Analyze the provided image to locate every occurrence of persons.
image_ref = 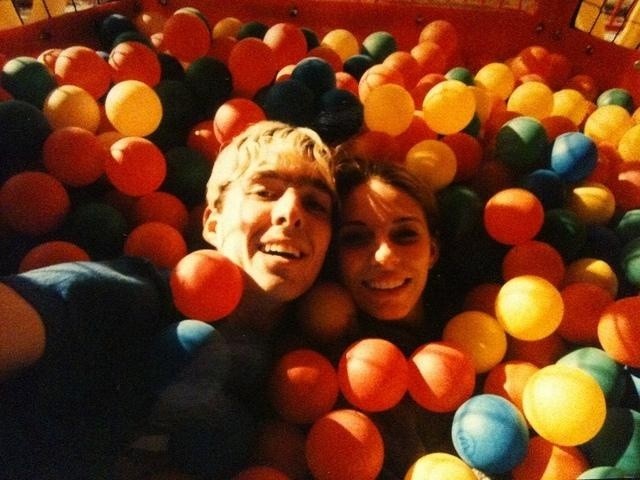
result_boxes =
[327,157,460,364]
[1,121,338,480]
